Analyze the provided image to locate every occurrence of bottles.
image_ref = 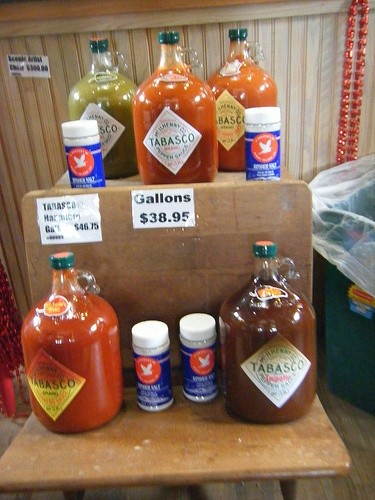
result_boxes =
[131,320,173,412]
[244,107,282,180]
[208,28,278,172]
[178,313,219,403]
[67,37,139,179]
[61,120,106,188]
[132,31,217,184]
[218,241,318,424]
[21,251,122,434]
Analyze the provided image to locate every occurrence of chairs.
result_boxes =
[0,180,352,500]
